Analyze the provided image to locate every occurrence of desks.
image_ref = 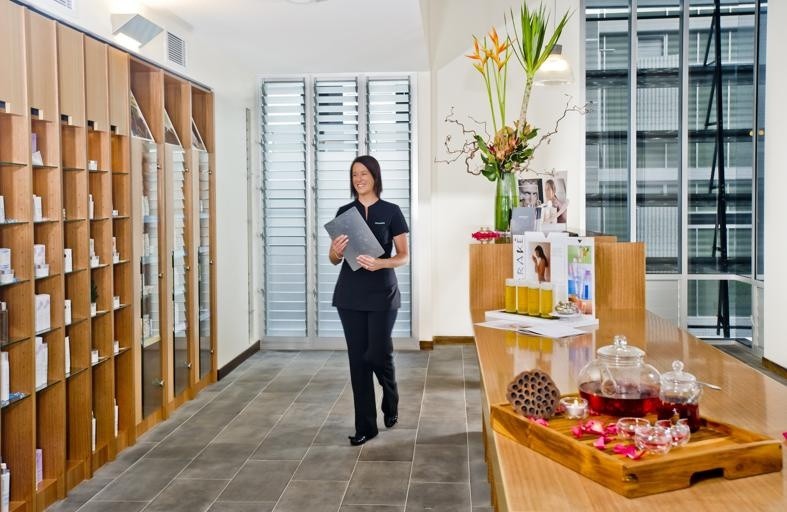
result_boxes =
[471,308,786,510]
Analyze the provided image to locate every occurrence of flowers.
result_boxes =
[434,3,596,181]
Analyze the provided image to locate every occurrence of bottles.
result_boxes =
[577,334,721,434]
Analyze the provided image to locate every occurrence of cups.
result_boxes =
[560,397,588,420]
[618,419,691,452]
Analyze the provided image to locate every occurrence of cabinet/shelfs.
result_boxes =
[1,1,216,511]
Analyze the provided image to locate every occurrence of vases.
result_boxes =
[496,172,518,244]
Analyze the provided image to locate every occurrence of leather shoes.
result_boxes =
[347,431,368,445]
[384,411,398,428]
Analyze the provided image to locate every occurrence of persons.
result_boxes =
[545,179,561,208]
[329,154,411,446]
[532,245,546,281]
[519,179,540,207]
